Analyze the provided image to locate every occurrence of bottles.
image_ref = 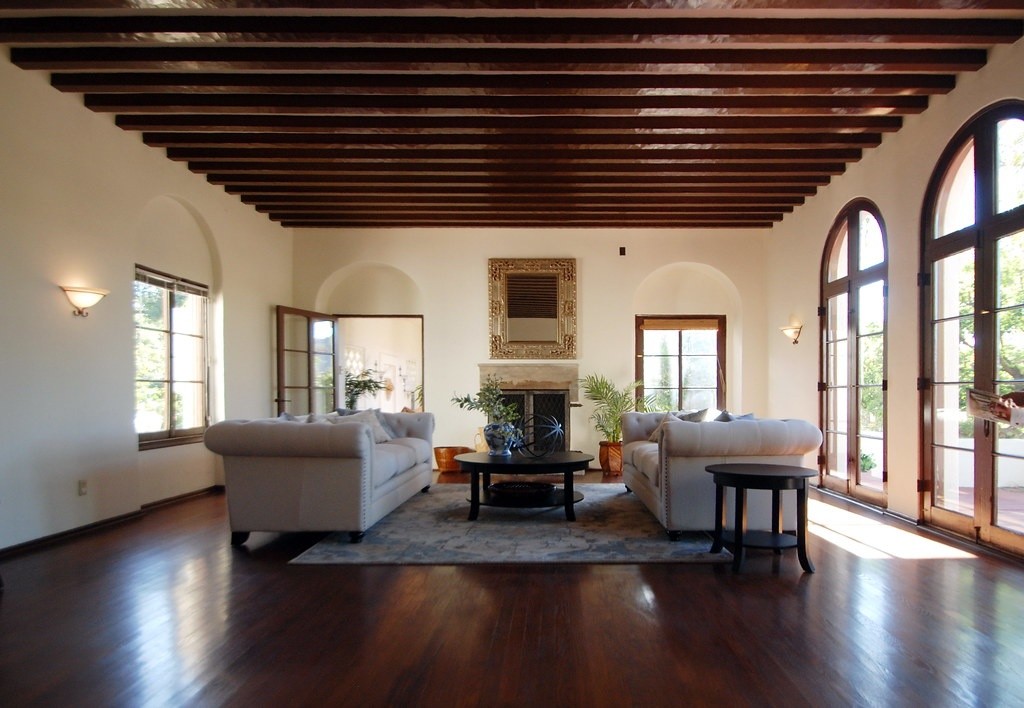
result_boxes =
[475,427,489,452]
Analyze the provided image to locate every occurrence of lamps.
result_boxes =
[58,284,110,318]
[778,326,805,345]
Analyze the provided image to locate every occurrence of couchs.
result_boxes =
[621,412,823,542]
[203,412,436,546]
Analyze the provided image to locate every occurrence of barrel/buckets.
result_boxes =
[434,447,468,471]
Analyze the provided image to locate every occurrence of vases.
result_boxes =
[484,414,516,456]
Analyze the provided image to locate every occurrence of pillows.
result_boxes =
[279,408,397,443]
[649,408,755,443]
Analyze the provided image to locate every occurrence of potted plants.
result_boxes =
[577,373,671,476]
[861,454,877,481]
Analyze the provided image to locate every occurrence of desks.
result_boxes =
[454,451,595,522]
[705,463,819,574]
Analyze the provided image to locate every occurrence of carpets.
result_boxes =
[288,483,733,564]
